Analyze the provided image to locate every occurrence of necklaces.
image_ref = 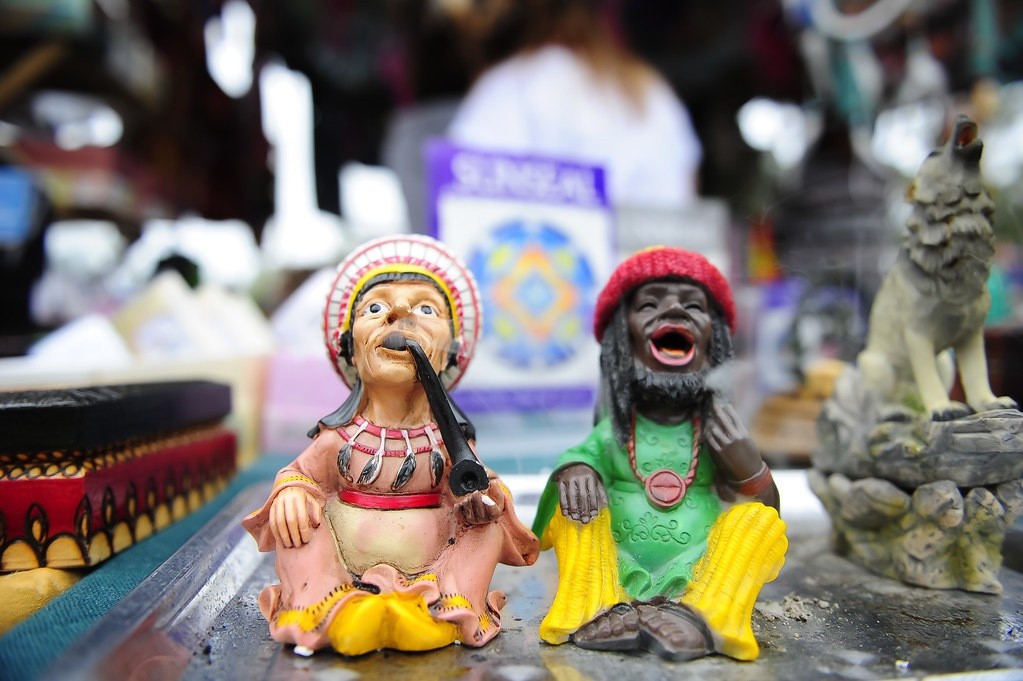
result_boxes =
[626,401,703,511]
[333,413,450,489]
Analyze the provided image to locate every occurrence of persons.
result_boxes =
[241,233,542,654]
[532,246,790,662]
[452,0,704,204]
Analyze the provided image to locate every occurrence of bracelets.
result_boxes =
[723,461,773,497]
